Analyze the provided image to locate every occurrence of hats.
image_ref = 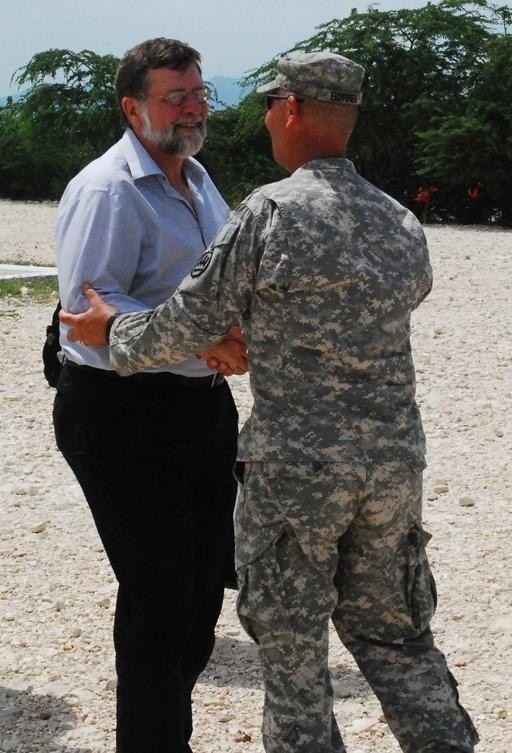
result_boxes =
[257,52,365,105]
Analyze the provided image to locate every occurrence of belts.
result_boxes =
[57,352,224,388]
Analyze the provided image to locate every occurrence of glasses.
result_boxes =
[141,85,215,105]
[264,91,306,110]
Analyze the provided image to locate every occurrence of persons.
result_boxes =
[42,34,248,752]
[57,51,481,753]
